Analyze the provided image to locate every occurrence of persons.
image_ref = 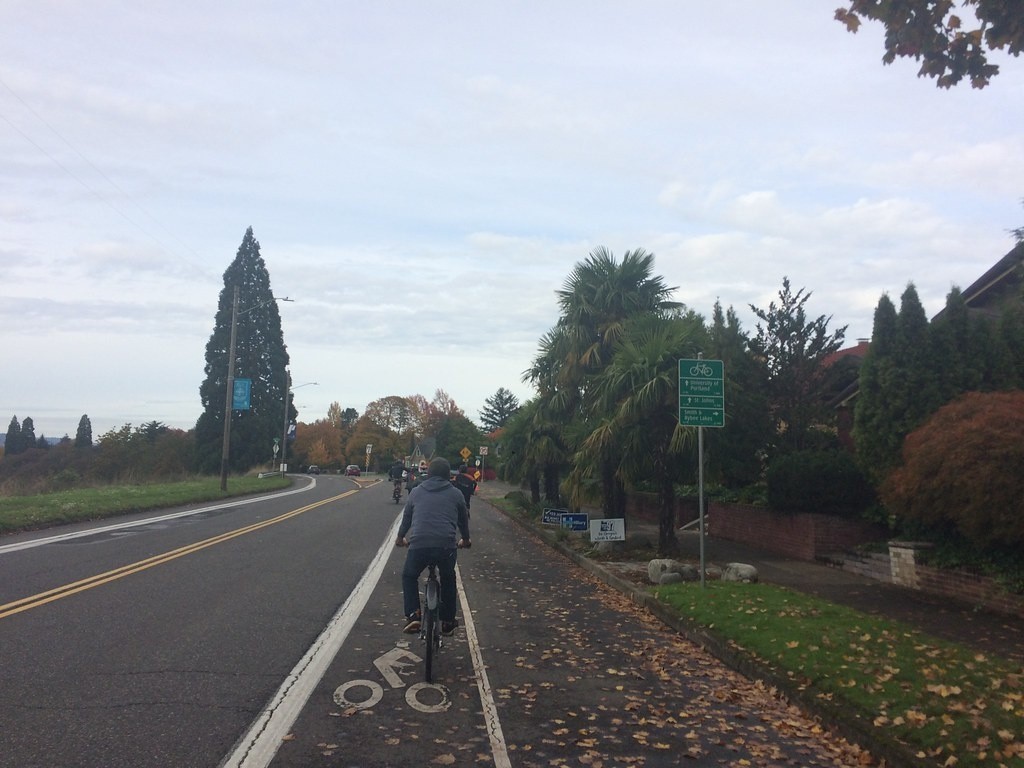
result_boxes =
[395,458,472,635]
[388,460,477,517]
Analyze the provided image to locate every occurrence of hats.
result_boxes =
[459,465,468,473]
[428,457,450,480]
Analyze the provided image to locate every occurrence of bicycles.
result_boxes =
[394,537,472,684]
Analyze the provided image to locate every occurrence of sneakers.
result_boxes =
[402,611,421,633]
[442,618,458,635]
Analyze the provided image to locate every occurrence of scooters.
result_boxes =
[388,475,407,504]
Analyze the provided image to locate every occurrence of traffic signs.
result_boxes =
[678,359,725,429]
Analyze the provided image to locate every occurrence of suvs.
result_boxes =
[307,465,320,476]
[344,465,361,477]
